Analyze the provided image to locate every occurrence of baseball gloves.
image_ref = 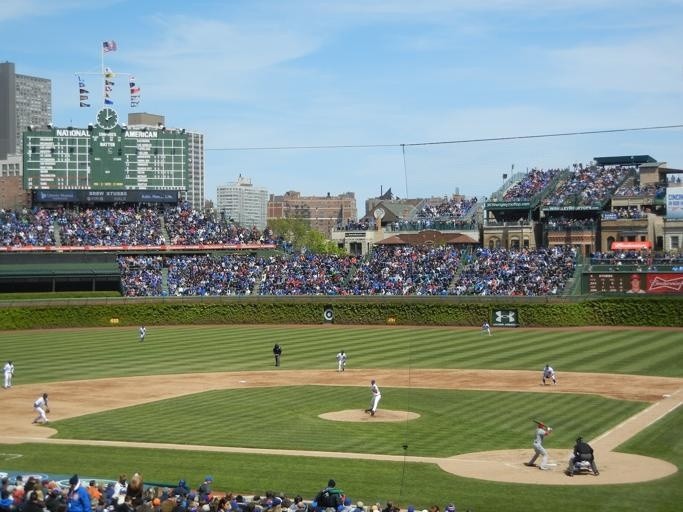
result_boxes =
[46,409,50,413]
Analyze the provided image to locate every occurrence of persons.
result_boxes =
[367,380,381,415]
[139,323,146,341]
[481,322,491,336]
[3,360,15,388]
[32,393,49,424]
[336,350,346,372]
[591,245,683,271]
[337,196,478,230]
[0,200,273,246]
[567,436,600,476]
[493,163,680,230]
[626,275,646,293]
[273,344,281,367]
[528,422,553,470]
[543,364,556,385]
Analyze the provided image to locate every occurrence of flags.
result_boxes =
[78,74,141,108]
[103,40,118,53]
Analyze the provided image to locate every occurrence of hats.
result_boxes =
[205,476,213,481]
[70,473,79,484]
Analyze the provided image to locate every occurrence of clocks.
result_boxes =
[95,106,119,131]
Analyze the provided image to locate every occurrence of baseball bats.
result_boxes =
[532,419,548,428]
